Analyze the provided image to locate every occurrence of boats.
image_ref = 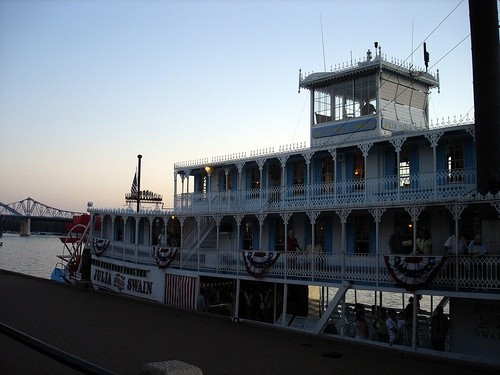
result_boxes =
[51,41,499,374]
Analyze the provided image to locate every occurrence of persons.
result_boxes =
[468,233,488,257]
[287,229,322,255]
[443,227,467,256]
[264,287,279,322]
[355,294,449,352]
[158,227,169,247]
[389,224,413,254]
[416,227,433,256]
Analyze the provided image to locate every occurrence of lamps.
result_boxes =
[171,215,175,219]
[355,169,358,175]
[256,179,258,184]
